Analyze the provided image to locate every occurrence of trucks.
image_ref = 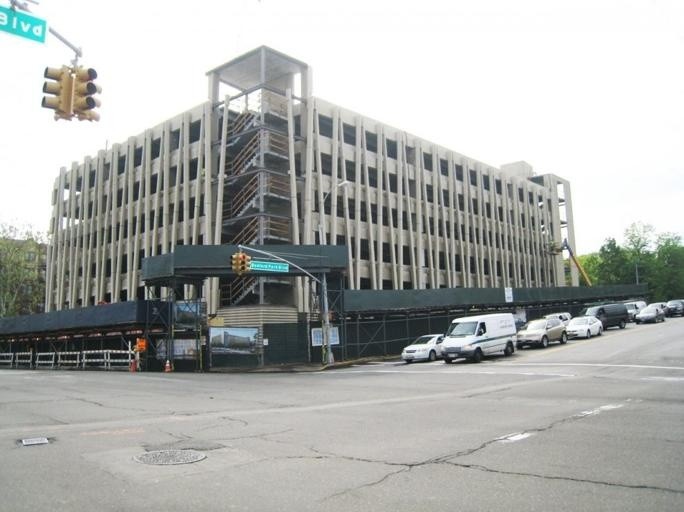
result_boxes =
[584,302,630,331]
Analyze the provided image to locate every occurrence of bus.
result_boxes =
[565,315,604,340]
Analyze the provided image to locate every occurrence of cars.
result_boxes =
[635,299,684,325]
[400,333,444,364]
[515,317,568,350]
[544,312,571,326]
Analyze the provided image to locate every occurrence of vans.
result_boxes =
[440,312,518,365]
[624,300,647,321]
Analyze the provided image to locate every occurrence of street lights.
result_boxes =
[318,179,352,365]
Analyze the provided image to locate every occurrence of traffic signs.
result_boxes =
[0,3,49,44]
[249,261,289,273]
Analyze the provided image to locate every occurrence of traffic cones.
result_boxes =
[164,358,173,373]
[129,358,138,373]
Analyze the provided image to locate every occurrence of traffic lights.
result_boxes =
[39,62,72,122]
[79,83,104,123]
[237,253,246,275]
[245,254,252,274]
[71,64,97,115]
[229,252,238,272]
